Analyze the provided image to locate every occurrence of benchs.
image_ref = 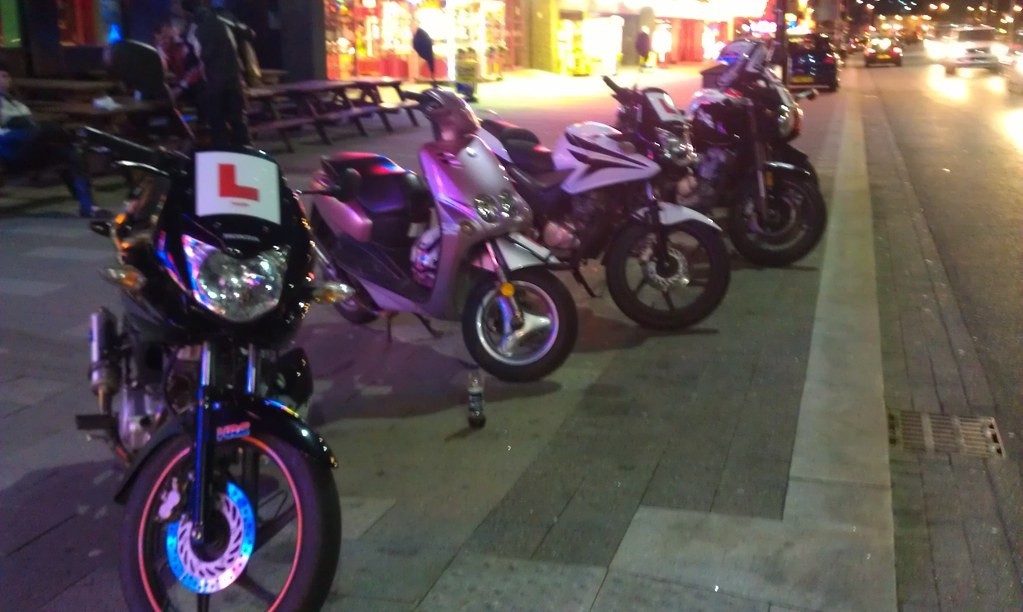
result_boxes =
[90,99,425,156]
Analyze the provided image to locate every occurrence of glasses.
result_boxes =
[689,88,735,144]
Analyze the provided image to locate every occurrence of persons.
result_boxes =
[150,0,257,154]
[637,25,653,69]
[0,69,115,218]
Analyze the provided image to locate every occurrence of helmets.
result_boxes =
[411,228,438,290]
[718,38,766,88]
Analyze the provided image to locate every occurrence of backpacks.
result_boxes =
[158,15,208,104]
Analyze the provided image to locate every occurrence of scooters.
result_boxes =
[308,27,578,384]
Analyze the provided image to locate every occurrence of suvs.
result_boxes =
[942,26,1008,77]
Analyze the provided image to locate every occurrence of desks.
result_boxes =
[11,73,404,183]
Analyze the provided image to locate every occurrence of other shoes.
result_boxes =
[80,204,114,220]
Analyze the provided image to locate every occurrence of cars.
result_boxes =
[784,35,843,94]
[862,35,904,69]
[832,27,936,65]
[1003,31,1023,94]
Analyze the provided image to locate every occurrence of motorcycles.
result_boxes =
[660,40,829,266]
[477,75,734,333]
[73,1,365,612]
